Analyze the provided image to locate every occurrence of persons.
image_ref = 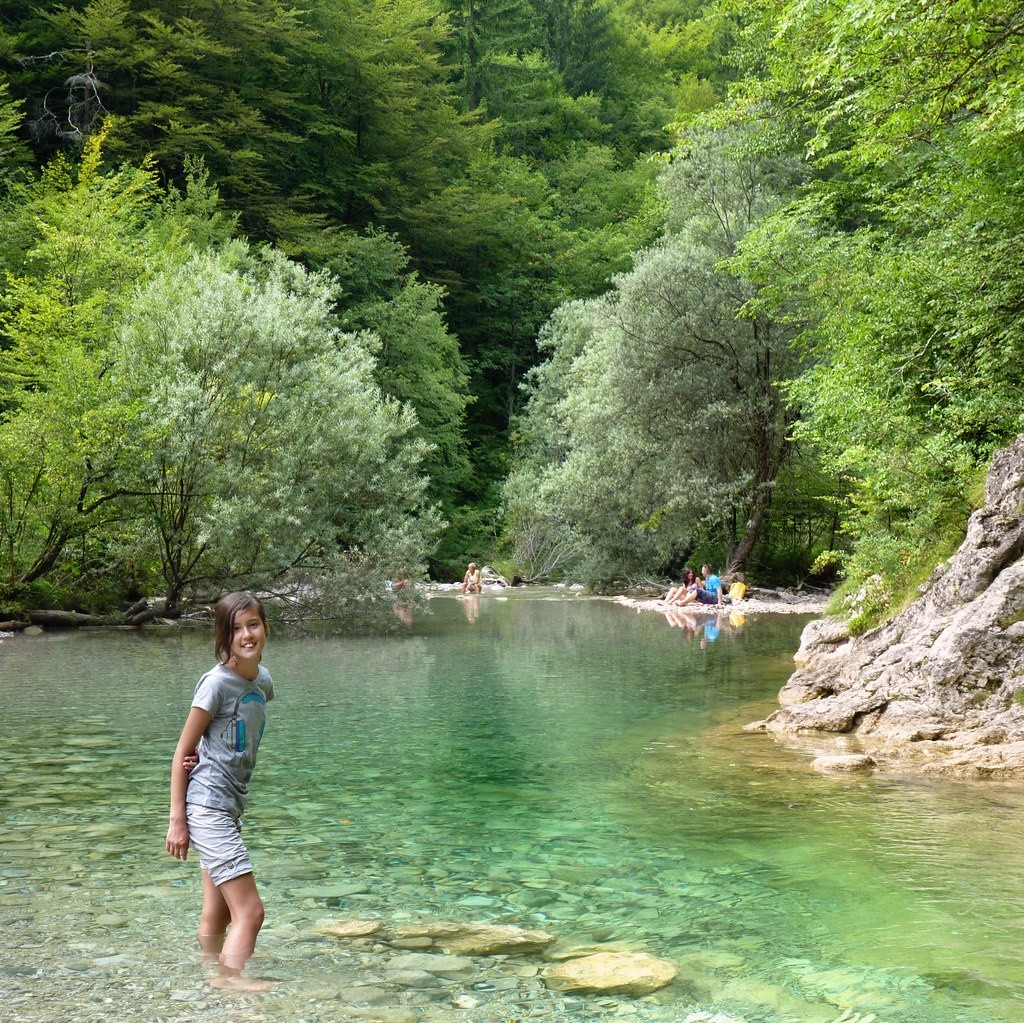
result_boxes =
[460,562,482,595]
[165,591,280,992]
[657,563,723,609]
[389,567,409,592]
